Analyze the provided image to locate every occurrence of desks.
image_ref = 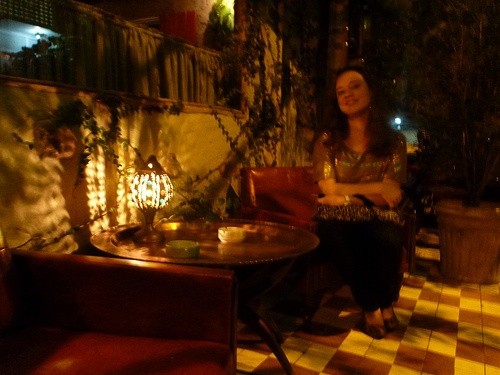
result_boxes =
[89,217,320,375]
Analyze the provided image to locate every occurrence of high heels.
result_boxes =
[362,307,398,338]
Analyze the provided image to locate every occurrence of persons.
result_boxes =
[310,63,408,339]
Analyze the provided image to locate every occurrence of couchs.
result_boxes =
[0,245,235,375]
[240,165,416,317]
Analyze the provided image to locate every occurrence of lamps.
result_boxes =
[128,156,174,243]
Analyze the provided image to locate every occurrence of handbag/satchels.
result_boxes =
[316,194,404,226]
